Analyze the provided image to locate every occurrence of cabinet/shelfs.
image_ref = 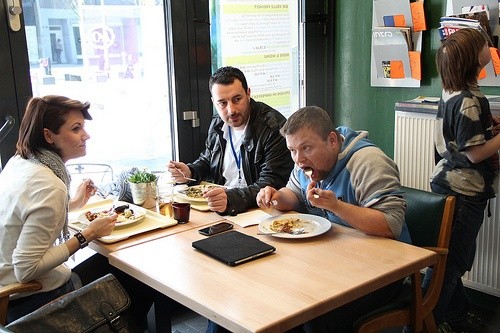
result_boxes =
[393,96,500,300]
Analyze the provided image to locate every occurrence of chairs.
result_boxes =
[354,186,458,333]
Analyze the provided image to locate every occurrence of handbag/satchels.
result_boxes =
[5,273,131,333]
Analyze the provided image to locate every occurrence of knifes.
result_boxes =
[266,199,277,206]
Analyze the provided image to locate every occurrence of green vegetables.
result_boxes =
[128,170,155,184]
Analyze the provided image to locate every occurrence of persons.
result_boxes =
[167,64,294,216]
[418,27,500,331]
[0,94,157,332]
[256,106,414,247]
[56,38,63,63]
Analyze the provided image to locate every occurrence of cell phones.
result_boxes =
[199,222,233,236]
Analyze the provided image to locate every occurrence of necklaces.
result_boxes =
[228,124,242,184]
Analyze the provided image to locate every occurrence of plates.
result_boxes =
[258,213,331,238]
[179,184,229,201]
[78,204,146,226]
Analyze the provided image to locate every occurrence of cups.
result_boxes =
[171,201,189,225]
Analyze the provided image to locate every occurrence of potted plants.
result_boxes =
[129,171,158,208]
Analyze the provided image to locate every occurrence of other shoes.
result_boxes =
[437,310,497,333]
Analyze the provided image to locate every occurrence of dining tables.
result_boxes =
[65,181,266,254]
[110,206,437,333]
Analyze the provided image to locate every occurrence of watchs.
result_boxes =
[74,232,89,249]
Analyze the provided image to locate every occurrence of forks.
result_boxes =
[172,165,197,183]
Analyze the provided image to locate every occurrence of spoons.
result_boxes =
[256,228,303,237]
[303,167,320,200]
